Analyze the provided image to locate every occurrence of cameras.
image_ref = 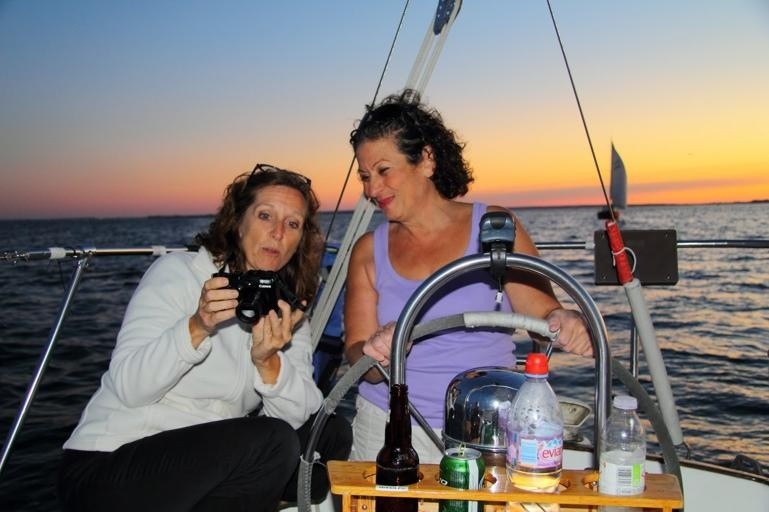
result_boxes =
[210,270,296,325]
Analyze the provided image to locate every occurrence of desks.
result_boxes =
[327,457,683,512]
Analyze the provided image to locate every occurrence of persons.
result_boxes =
[61,164,352,512]
[344,88,595,464]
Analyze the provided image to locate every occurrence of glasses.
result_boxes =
[244,164,311,197]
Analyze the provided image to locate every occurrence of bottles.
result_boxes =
[596,395,646,512]
[375,382,419,512]
[504,350,564,512]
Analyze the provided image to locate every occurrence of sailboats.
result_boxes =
[598,142,627,220]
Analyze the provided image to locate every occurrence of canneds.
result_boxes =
[438,442,485,512]
